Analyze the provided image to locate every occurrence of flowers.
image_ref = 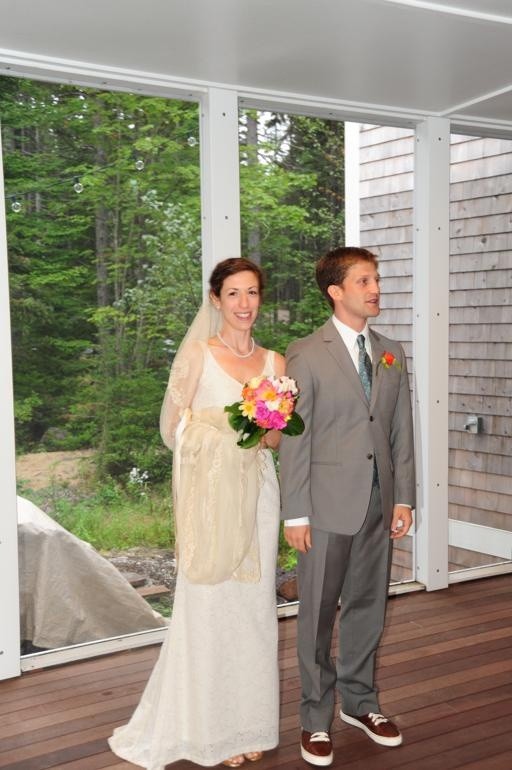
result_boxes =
[223,374,306,447]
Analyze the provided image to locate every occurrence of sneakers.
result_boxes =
[300,730,335,767]
[338,705,402,748]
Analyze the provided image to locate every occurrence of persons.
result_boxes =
[107,259,296,770]
[276,247,418,767]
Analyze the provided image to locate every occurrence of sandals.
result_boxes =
[222,752,262,767]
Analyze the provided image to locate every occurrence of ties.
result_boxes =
[354,335,372,400]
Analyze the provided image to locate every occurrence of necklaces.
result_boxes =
[216,331,260,361]
